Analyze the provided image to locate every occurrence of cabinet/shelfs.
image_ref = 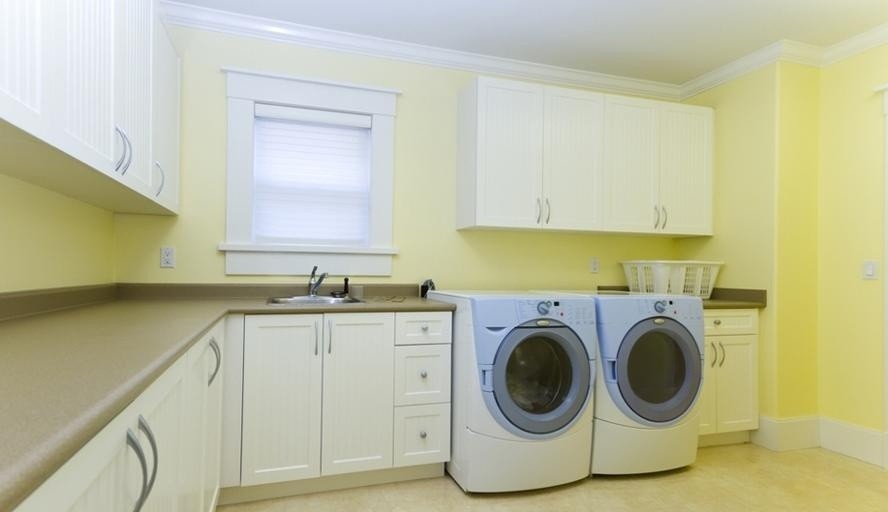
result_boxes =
[0,0,153,201]
[10,359,184,512]
[239,311,394,487]
[394,311,452,469]
[454,75,601,236]
[602,94,713,236]
[185,318,226,512]
[699,308,759,437]
[153,13,181,215]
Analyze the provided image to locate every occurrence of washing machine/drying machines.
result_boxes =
[594,290,704,478]
[427,291,596,494]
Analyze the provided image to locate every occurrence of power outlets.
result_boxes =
[159,247,177,268]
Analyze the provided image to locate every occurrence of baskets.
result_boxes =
[618,258,725,301]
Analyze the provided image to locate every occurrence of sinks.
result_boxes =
[265,295,365,305]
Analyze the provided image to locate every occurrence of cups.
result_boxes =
[349,285,364,300]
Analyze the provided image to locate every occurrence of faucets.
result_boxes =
[307,264,328,295]
